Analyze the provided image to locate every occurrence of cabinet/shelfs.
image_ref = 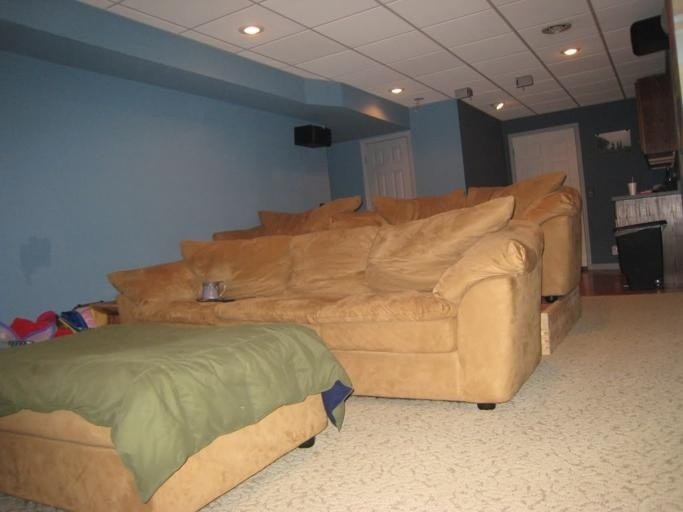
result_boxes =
[634,73,678,170]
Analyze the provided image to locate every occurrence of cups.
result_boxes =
[628,181,637,196]
[202,280,226,299]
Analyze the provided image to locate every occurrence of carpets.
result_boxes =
[3,292,683,512]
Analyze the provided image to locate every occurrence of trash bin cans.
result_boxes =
[612,220,667,290]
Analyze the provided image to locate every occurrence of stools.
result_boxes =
[0,318,356,512]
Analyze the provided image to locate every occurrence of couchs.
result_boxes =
[213,186,584,303]
[94,220,543,410]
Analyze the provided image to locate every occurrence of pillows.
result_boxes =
[178,173,567,298]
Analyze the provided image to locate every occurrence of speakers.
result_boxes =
[294,125,331,147]
